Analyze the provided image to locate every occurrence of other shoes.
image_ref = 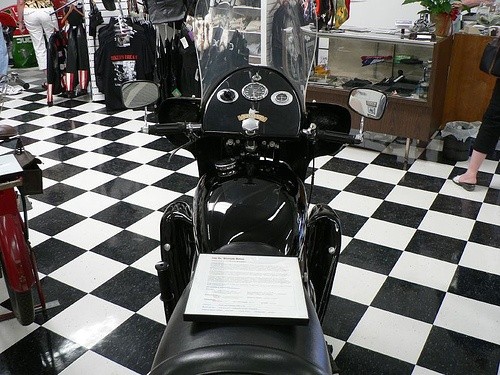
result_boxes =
[42,82,48,89]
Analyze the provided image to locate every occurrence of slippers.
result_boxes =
[452,175,475,191]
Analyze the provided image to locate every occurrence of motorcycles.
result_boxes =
[120,0,389,375]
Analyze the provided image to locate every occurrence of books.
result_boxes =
[183,253,310,326]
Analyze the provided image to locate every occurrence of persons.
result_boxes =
[18,0,60,90]
[452,37,500,191]
[0,21,24,96]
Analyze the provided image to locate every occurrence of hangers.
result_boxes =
[49,0,300,57]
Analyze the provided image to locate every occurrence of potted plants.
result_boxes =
[401,0,471,37]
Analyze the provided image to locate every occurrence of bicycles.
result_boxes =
[0,124,48,326]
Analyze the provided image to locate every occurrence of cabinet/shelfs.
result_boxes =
[439,32,500,139]
[301,23,453,142]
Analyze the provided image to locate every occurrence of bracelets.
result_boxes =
[18,20,23,24]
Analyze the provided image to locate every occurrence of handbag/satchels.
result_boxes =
[479,36,500,77]
